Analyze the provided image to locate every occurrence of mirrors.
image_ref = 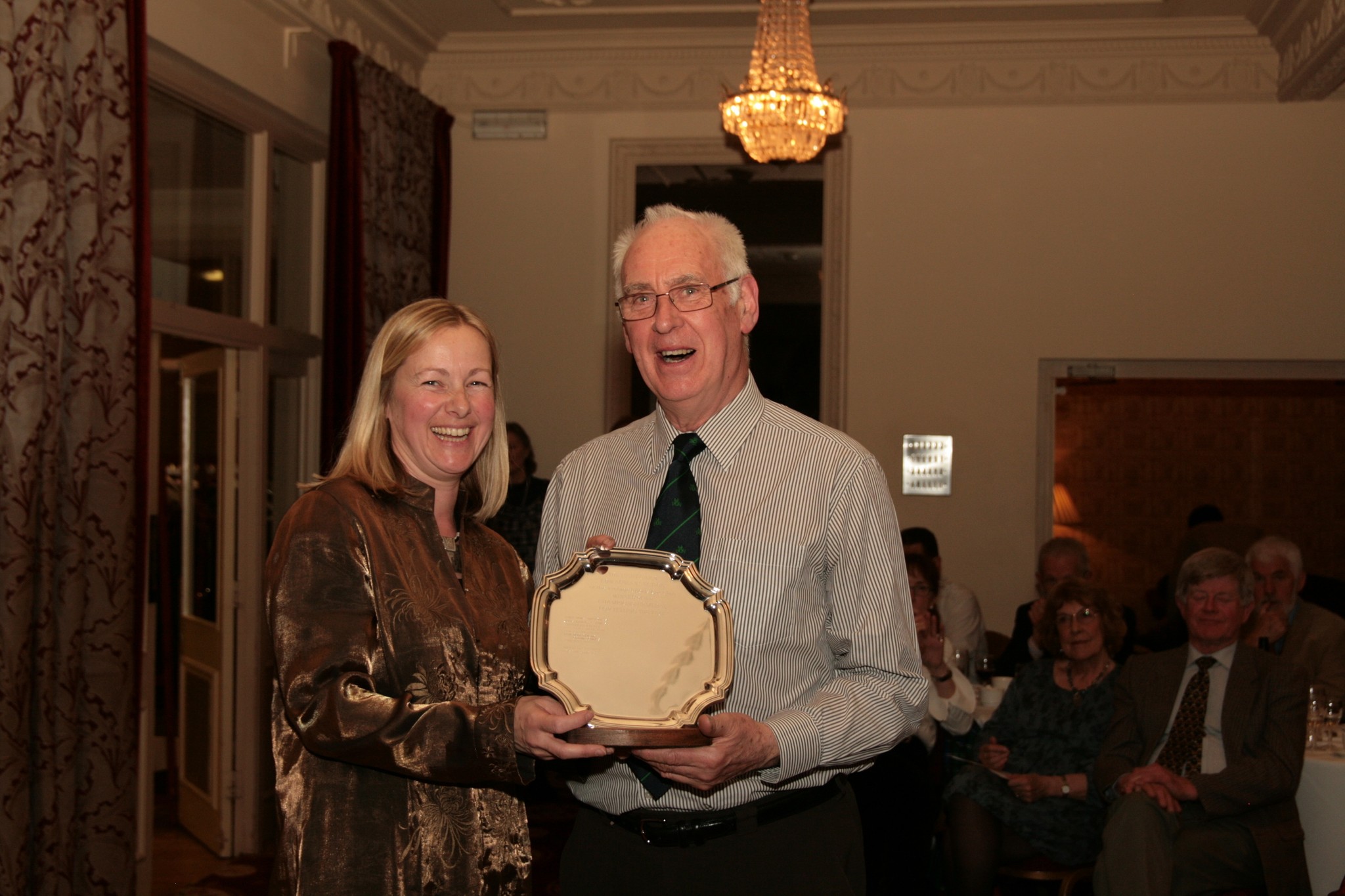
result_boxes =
[1033,356,1344,629]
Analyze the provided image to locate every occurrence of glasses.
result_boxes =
[614,276,739,322]
[1053,607,1097,626]
[908,583,932,596]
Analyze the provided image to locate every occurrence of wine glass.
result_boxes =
[974,652,997,688]
[948,647,970,677]
[1306,683,1343,756]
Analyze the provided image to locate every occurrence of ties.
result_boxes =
[625,433,706,801]
[1155,657,1218,778]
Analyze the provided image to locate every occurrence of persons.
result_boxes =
[264,295,615,894]
[469,417,554,576]
[885,499,1343,896]
[532,204,929,896]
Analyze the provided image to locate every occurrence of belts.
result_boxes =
[603,778,841,848]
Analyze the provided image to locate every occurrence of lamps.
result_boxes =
[718,0,848,164]
